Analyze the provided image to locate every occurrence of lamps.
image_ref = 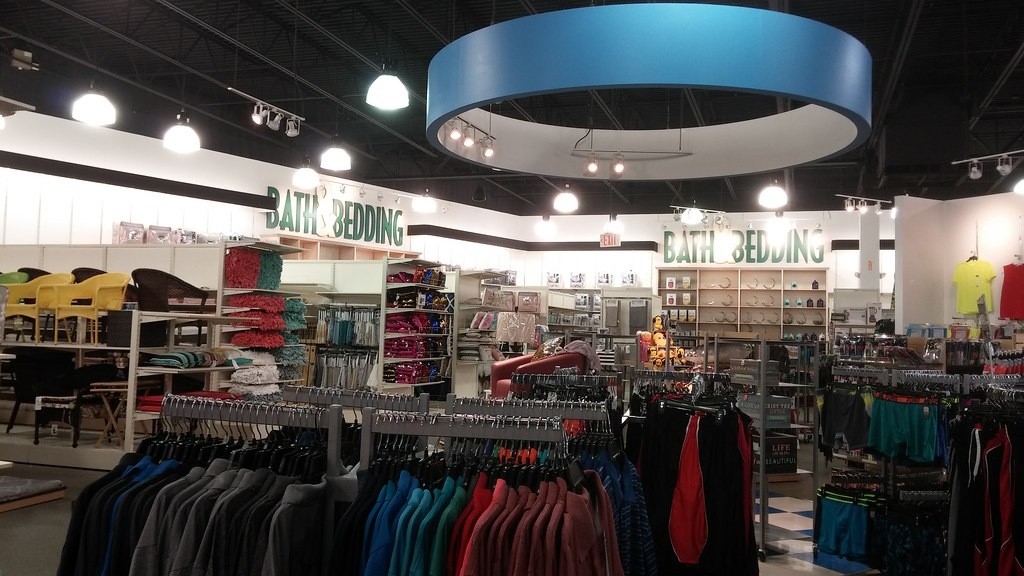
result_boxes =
[65,56,1023,232]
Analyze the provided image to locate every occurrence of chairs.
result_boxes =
[0,265,211,451]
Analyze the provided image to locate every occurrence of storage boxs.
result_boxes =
[698,328,759,348]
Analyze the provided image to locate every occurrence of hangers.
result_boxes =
[152,337,1020,509]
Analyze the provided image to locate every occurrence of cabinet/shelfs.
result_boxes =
[281,256,449,412]
[654,266,830,362]
[214,236,309,432]
[442,268,550,406]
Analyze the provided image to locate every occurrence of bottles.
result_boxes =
[812,280,818,289]
[811,332,817,341]
[791,280,798,289]
[802,333,809,340]
[797,297,802,307]
[806,298,813,307]
[789,332,794,340]
[796,331,801,340]
[785,297,790,307]
[818,332,826,341]
[784,332,788,339]
[817,297,823,307]
